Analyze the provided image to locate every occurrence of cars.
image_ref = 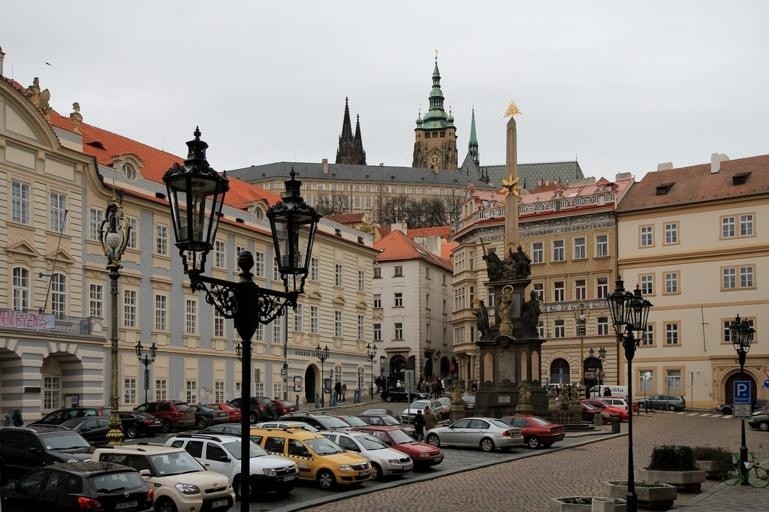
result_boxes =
[423,416,525,452]
[500,415,565,450]
[25,395,295,448]
[381,387,475,423]
[715,400,768,430]
[249,408,442,492]
[559,385,639,425]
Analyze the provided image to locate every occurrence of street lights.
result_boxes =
[134,340,158,409]
[89,178,135,416]
[588,347,607,396]
[365,343,378,400]
[604,279,658,504]
[315,344,330,408]
[722,312,759,491]
[163,127,329,511]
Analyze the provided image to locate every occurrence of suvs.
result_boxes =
[161,431,300,501]
[1,460,154,512]
[1,423,98,486]
[638,394,686,412]
[90,442,236,512]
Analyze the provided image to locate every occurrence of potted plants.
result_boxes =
[551,444,736,512]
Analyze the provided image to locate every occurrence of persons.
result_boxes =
[314,393,320,409]
[294,395,300,411]
[340,383,348,402]
[371,364,457,402]
[471,245,542,337]
[413,409,426,441]
[334,381,342,402]
[12,408,24,428]
[423,405,438,431]
[604,387,612,397]
